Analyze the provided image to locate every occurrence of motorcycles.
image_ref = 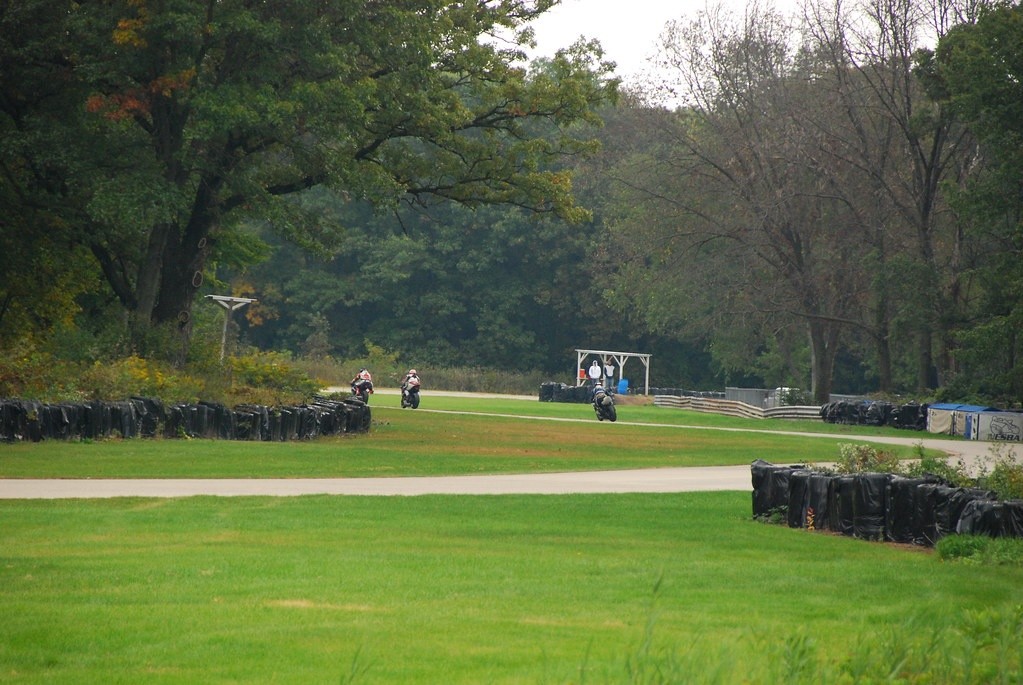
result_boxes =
[350,383,369,405]
[400,385,420,409]
[591,394,617,422]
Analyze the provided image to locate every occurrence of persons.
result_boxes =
[604,361,615,387]
[592,382,611,411]
[589,360,601,386]
[351,368,374,396]
[401,369,420,396]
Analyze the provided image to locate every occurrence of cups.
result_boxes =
[580,369,585,378]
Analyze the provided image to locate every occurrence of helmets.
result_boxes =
[409,370,416,374]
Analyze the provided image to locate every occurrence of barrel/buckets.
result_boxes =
[618,379,629,395]
